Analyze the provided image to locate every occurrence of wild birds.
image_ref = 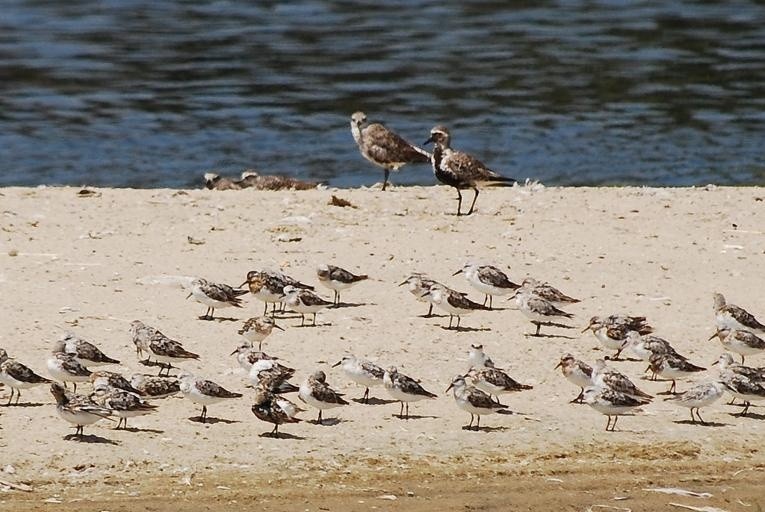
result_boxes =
[392,259,584,340]
[46,311,441,441]
[423,125,525,216]
[441,341,539,433]
[0,349,53,407]
[178,257,375,329]
[350,111,432,191]
[202,169,327,191]
[553,289,764,432]
